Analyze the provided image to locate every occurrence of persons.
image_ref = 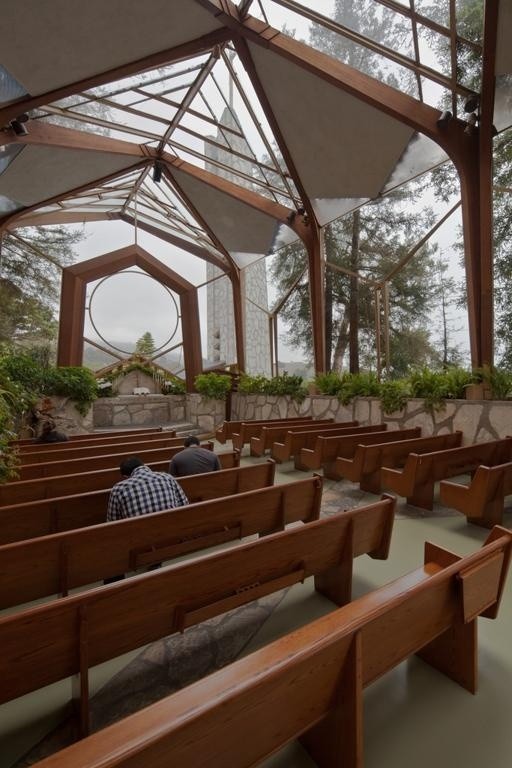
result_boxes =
[103,456,189,585]
[36,421,66,443]
[169,435,223,477]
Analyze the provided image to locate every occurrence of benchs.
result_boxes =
[1,416,511,766]
